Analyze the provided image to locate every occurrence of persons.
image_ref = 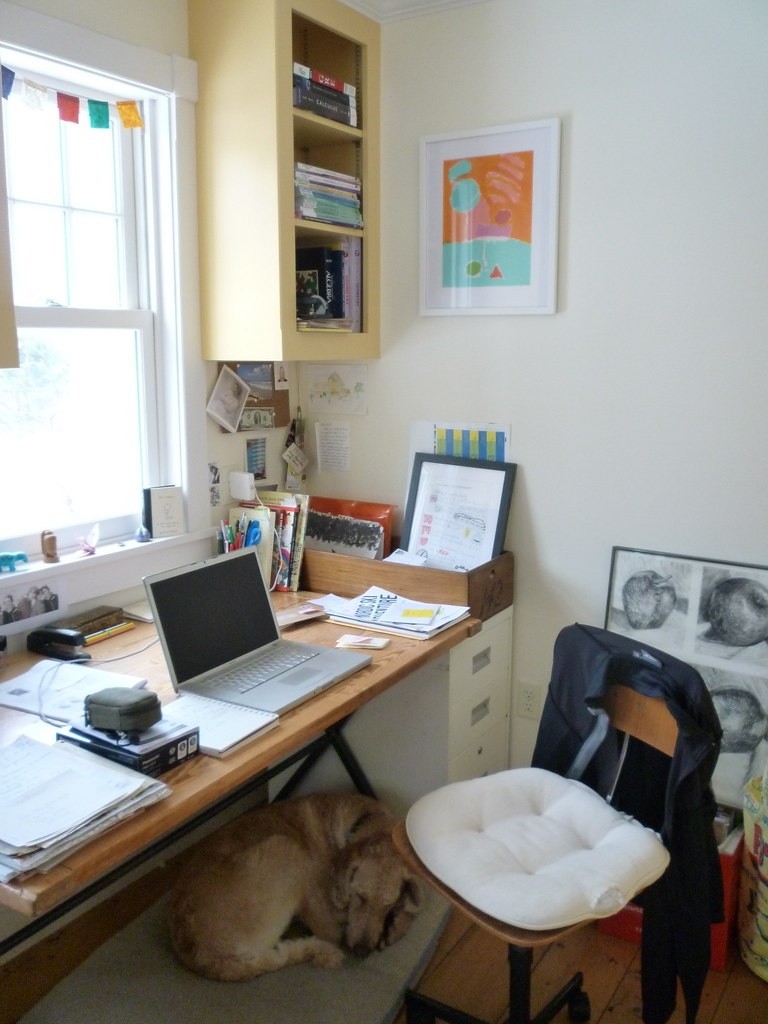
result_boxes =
[1,586,59,626]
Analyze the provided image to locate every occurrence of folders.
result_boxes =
[308,494,398,559]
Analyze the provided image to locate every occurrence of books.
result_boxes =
[293,62,358,128]
[295,161,364,228]
[229,489,312,592]
[0,734,175,886]
[159,693,280,759]
[0,658,149,722]
[296,237,362,332]
[324,582,471,640]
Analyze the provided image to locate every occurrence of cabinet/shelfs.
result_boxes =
[188,0,382,364]
[268,605,515,822]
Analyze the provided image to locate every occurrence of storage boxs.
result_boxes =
[596,826,743,967]
[56,712,200,780]
[303,549,519,617]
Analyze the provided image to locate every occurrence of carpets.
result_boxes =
[21,860,449,1024]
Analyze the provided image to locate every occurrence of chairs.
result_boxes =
[400,624,709,1024]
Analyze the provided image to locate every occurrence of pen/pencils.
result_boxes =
[216,513,261,555]
[84,622,135,645]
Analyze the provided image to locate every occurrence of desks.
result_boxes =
[0,587,515,918]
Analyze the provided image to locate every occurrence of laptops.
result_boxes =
[142,544,373,717]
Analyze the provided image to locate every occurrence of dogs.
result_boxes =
[167,792,425,982]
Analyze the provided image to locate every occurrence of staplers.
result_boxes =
[26,628,92,664]
